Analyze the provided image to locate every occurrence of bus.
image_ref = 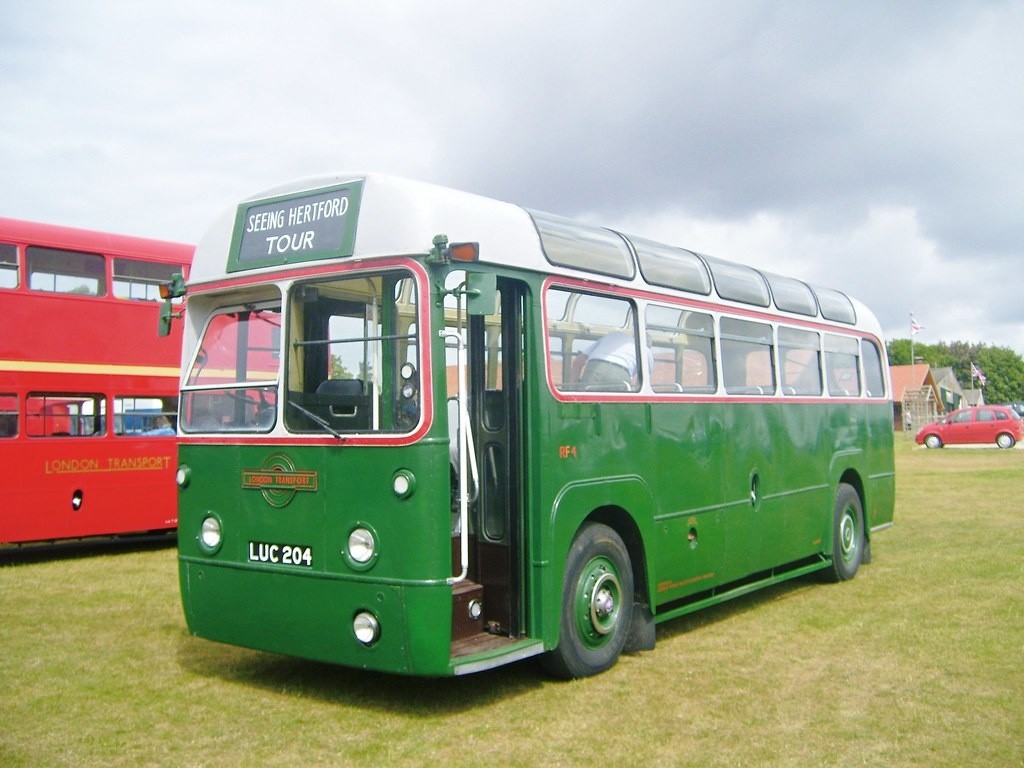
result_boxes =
[0,215,333,552]
[157,169,896,679]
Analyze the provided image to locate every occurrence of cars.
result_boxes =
[914,405,1023,450]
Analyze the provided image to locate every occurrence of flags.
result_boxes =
[911,313,922,336]
[970,363,985,385]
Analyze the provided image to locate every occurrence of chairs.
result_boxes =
[553,381,873,397]
[313,378,372,396]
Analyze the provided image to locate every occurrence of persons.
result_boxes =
[572,331,654,392]
